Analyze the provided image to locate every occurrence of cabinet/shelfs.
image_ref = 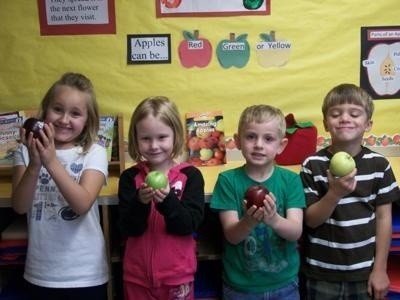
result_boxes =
[1,155,400,299]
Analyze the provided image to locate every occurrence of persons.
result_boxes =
[11,71,112,300]
[300,84,400,300]
[117,94,205,300]
[209,105,307,299]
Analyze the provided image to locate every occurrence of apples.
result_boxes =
[145,170,168,191]
[161,0,182,8]
[247,186,269,209]
[187,119,236,167]
[23,118,48,144]
[215,32,250,69]
[329,151,356,177]
[367,42,400,96]
[276,113,318,165]
[255,31,292,68]
[317,137,331,149]
[178,29,212,68]
[242,0,264,10]
[366,135,400,146]
